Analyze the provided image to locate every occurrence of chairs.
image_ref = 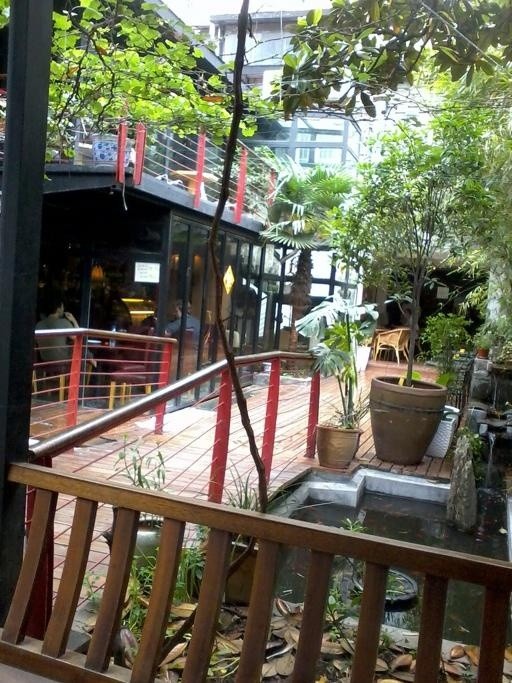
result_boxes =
[374,328,411,365]
[31,321,216,412]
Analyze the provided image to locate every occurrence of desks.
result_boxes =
[171,171,217,201]
[369,329,395,362]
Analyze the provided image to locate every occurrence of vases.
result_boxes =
[460,342,466,349]
[91,132,135,169]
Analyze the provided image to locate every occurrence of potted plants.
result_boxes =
[475,332,497,360]
[190,458,336,610]
[104,431,174,580]
[316,101,500,468]
[261,147,380,471]
[402,371,463,460]
[327,517,420,615]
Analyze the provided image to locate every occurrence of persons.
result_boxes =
[36,294,99,404]
[162,300,204,362]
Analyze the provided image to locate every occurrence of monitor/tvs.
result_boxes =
[132,259,161,286]
